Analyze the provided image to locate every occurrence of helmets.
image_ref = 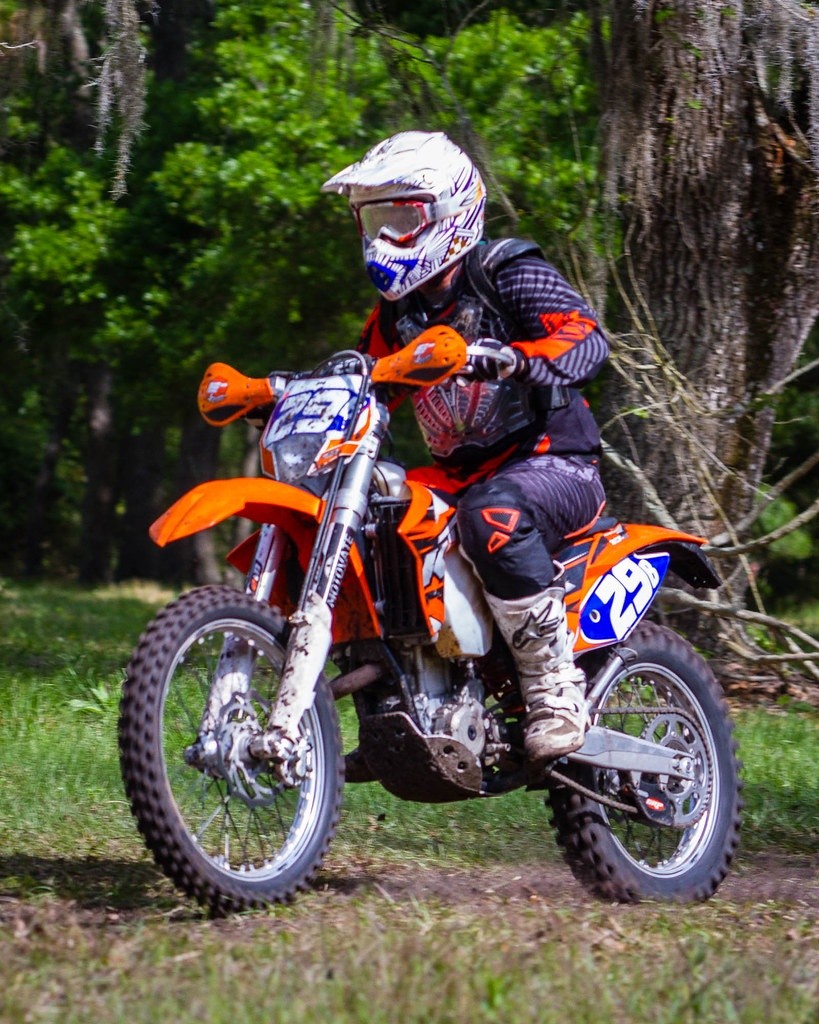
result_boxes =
[320,131,487,302]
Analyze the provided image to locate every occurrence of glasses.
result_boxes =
[350,169,484,245]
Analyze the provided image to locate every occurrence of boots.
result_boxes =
[344,745,374,781]
[481,561,592,767]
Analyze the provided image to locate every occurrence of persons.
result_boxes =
[244,132,606,758]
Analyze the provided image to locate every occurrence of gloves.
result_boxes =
[453,337,526,386]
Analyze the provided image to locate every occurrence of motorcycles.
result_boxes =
[119,325,744,916]
